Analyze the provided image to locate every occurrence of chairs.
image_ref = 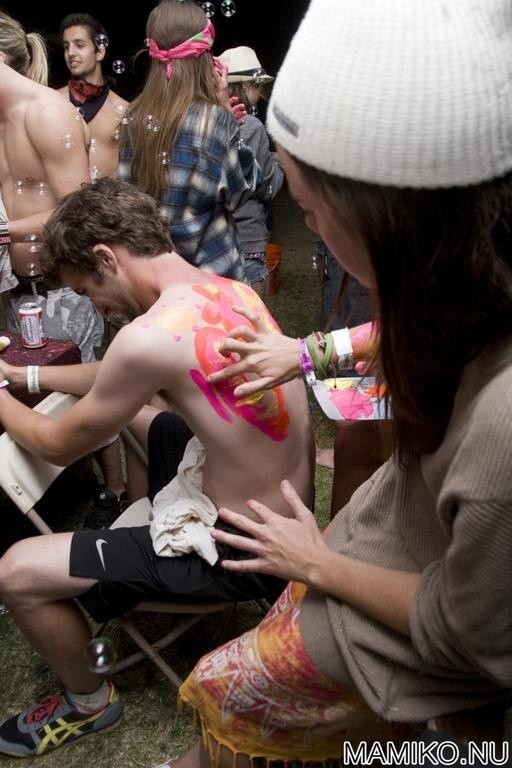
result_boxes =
[0,389,273,693]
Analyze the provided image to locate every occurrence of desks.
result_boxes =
[0,330,101,499]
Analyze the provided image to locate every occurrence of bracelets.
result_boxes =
[296,326,355,386]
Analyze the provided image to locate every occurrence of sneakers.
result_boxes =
[81,484,130,530]
[0,677,122,760]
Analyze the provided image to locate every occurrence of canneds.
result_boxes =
[18,302,47,349]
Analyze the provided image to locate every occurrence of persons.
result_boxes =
[0,174,316,757]
[155,0,510,767]
[0,2,286,530]
[317,246,397,520]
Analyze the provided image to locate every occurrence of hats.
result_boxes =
[217,46,274,85]
[261,0,512,191]
